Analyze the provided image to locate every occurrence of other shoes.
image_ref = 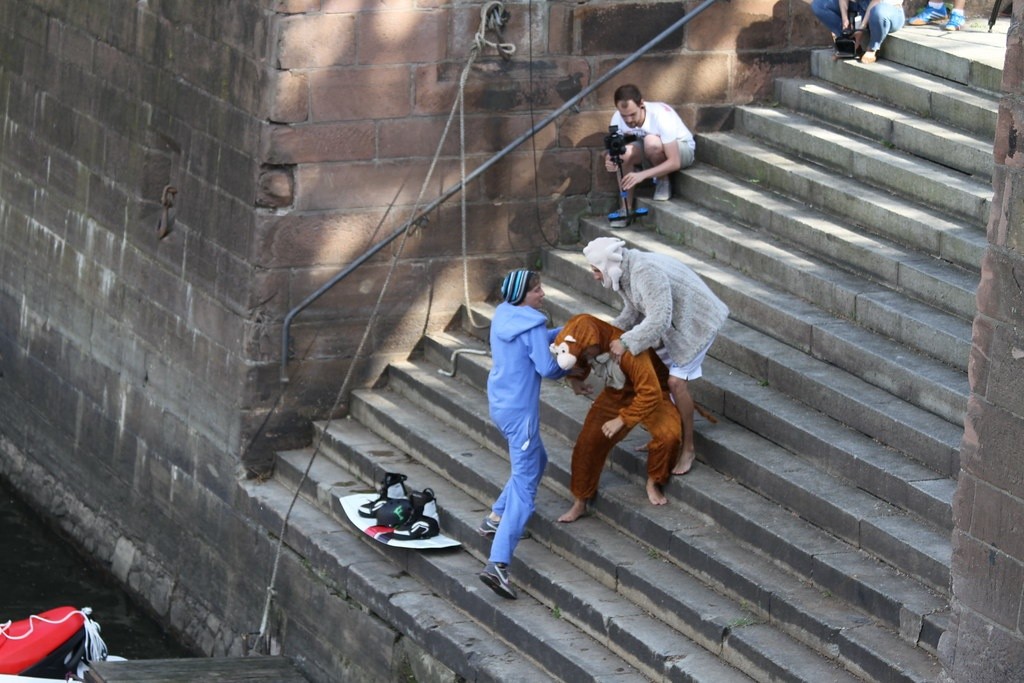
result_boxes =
[862,56,876,65]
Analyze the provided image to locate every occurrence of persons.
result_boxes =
[583,237,729,475]
[603,84,697,228]
[811,0,906,63]
[479,268,566,600]
[550,312,719,523]
[907,0,967,31]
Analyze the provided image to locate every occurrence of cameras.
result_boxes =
[603,124,637,157]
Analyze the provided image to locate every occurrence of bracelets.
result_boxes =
[620,339,629,349]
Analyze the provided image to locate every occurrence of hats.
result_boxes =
[583,236,626,292]
[500,270,529,305]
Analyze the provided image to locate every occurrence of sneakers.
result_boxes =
[479,563,518,600]
[358,472,409,518]
[393,487,440,540]
[610,220,629,228]
[907,2,948,25]
[945,11,965,31]
[653,179,671,201]
[479,517,531,540]
